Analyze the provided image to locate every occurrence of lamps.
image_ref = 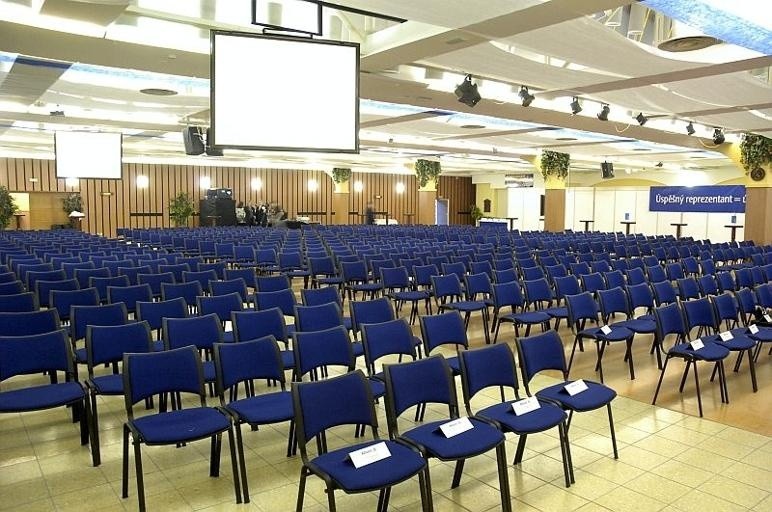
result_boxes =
[454,73,727,147]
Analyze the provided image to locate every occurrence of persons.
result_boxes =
[236,201,269,226]
[366,203,375,224]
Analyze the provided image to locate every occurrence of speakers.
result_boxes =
[182,127,204,155]
[601,163,615,179]
[206,128,223,156]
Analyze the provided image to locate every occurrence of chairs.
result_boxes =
[420,311,505,422]
[381,353,509,512]
[514,329,618,484]
[83,320,181,466]
[652,303,725,417]
[0,328,99,468]
[361,318,453,436]
[213,335,298,502]
[0,204,422,398]
[679,298,757,404]
[424,206,772,365]
[291,325,384,455]
[458,343,571,512]
[290,369,428,511]
[120,344,242,512]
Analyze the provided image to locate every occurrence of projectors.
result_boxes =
[50,111,64,121]
